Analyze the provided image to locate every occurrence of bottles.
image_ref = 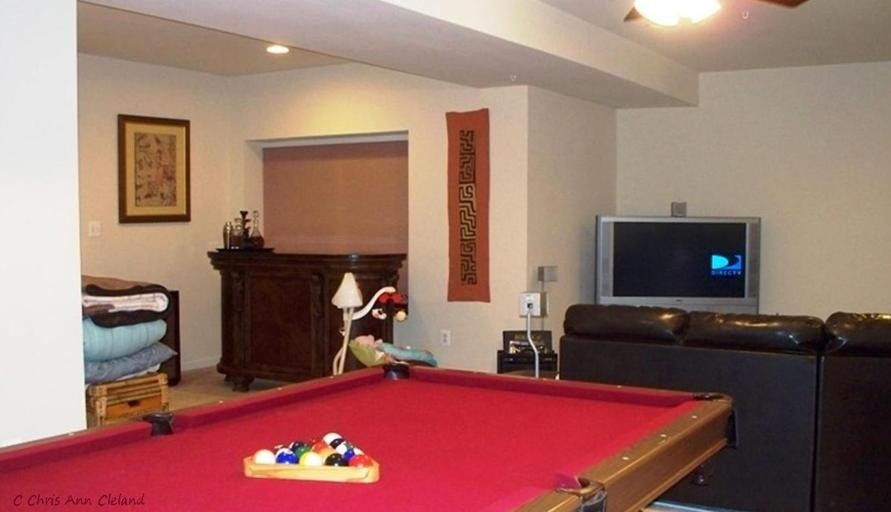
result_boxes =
[249,211,265,250]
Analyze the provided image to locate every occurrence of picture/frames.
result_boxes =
[117,113,191,224]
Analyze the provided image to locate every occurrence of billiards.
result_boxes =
[251,431,374,467]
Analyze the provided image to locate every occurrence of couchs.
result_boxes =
[558,302,890,512]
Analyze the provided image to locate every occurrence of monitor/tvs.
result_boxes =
[594,215,762,316]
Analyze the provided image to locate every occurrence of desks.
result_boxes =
[85,371,170,428]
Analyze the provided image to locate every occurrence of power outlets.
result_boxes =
[439,329,451,347]
[518,292,541,316]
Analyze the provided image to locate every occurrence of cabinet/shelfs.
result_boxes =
[206,250,409,393]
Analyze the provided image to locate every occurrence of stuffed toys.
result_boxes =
[371,291,407,322]
